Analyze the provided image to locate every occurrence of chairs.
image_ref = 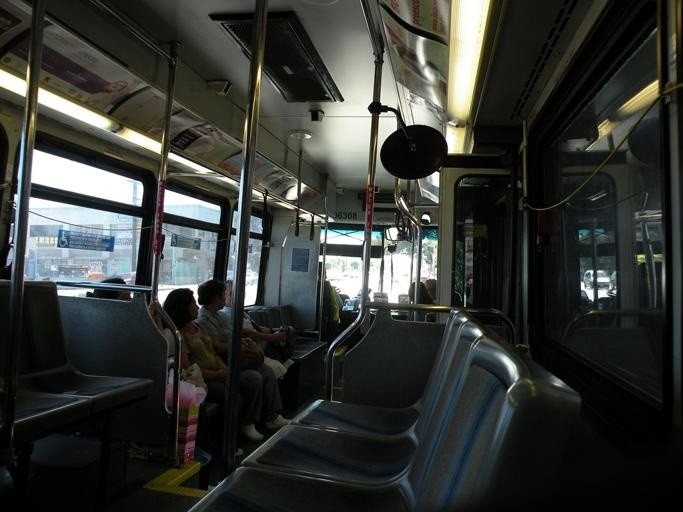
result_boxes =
[188,282,581,511]
[0,282,333,493]
[562,307,665,361]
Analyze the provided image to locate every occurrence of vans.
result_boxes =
[86,272,106,283]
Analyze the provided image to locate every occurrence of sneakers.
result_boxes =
[265,414,292,429]
[244,424,264,440]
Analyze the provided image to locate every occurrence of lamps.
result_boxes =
[583,76,659,153]
[0,68,296,213]
[445,121,467,153]
[443,0,492,128]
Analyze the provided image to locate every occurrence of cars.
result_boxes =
[583,269,610,289]
[119,270,137,292]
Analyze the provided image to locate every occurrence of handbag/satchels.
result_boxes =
[268,326,297,364]
[239,341,263,371]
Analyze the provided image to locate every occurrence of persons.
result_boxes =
[316,260,344,311]
[161,287,265,442]
[422,278,437,302]
[83,277,166,336]
[190,278,294,434]
[212,280,301,418]
[406,281,432,322]
